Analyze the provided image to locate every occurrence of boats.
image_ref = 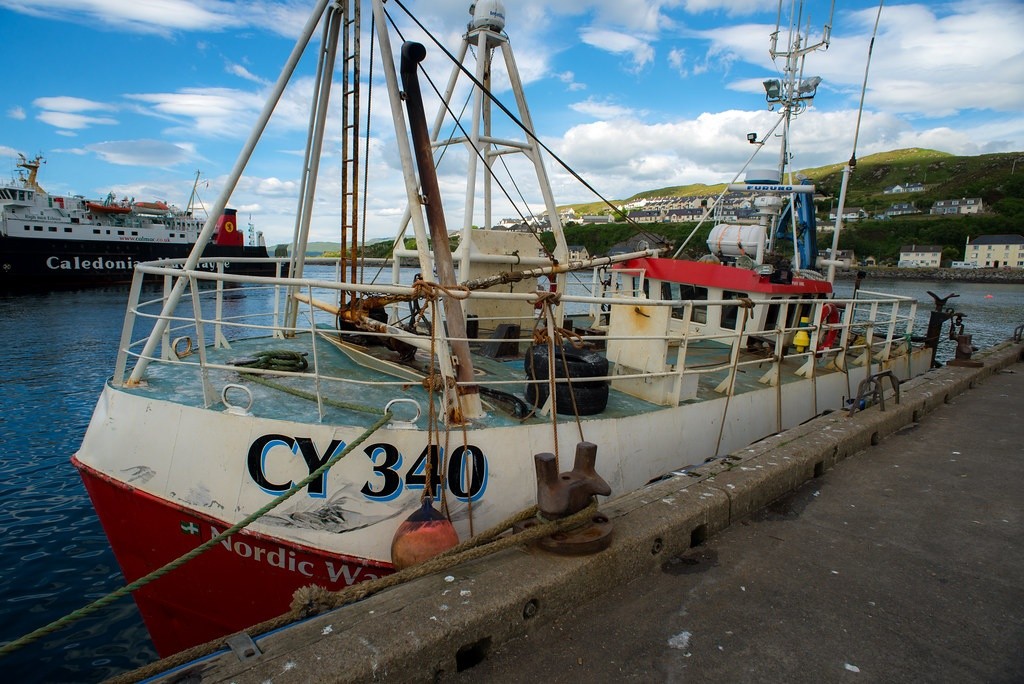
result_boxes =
[70,1,953,662]
[0,148,297,295]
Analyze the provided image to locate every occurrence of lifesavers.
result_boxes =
[814,304,838,357]
